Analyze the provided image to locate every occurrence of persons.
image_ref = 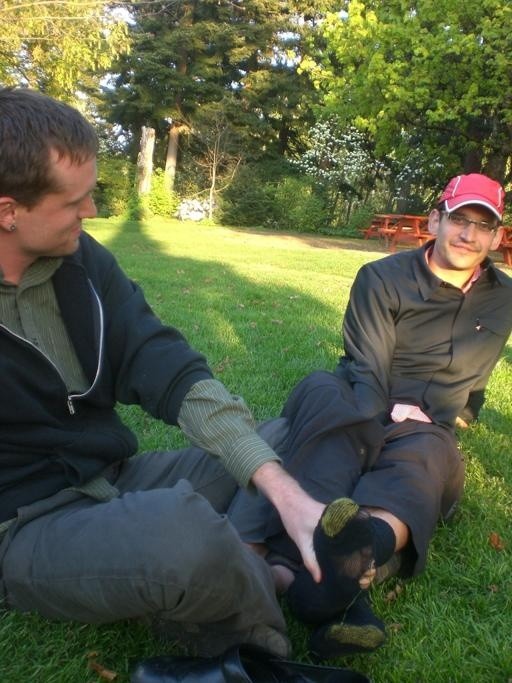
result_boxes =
[1,85,377,660]
[265,172,512,661]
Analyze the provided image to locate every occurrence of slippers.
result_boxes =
[127,641,374,683]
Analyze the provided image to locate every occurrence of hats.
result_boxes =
[438,173,507,223]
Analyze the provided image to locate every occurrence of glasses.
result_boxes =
[442,212,498,233]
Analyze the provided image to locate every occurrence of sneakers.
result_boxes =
[224,623,293,660]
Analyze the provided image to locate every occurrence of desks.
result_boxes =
[376,214,390,248]
[390,215,428,251]
[496,226,512,262]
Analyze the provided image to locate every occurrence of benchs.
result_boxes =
[359,222,434,265]
[503,244,512,266]
